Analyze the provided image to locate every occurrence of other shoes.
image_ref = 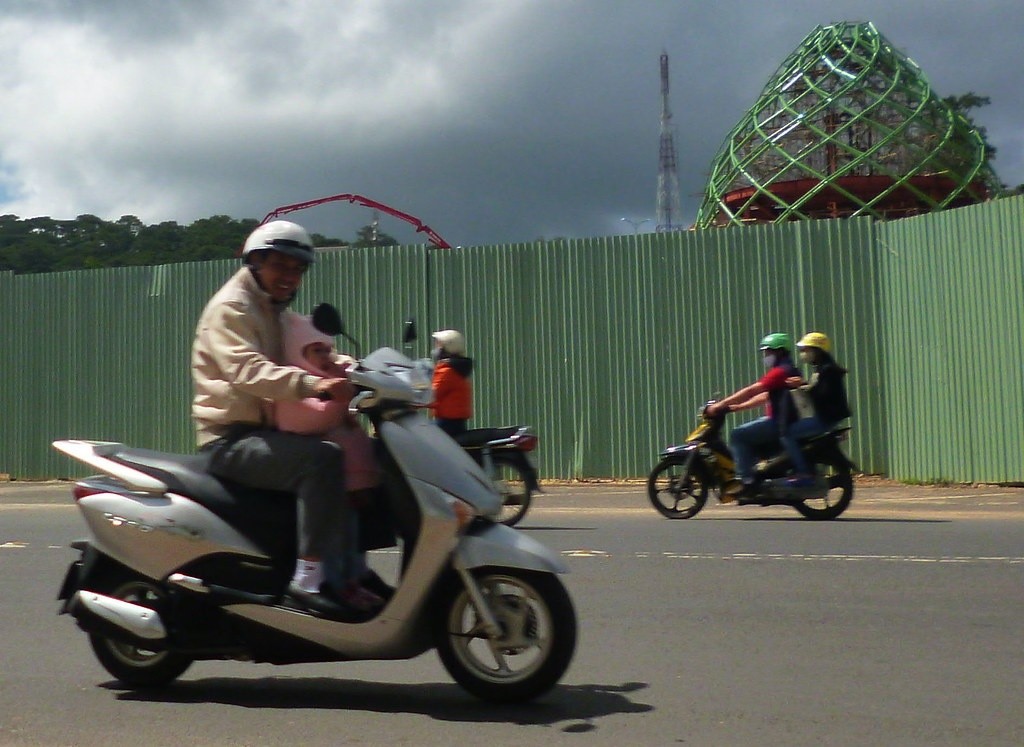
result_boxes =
[783,474,816,487]
[727,483,749,497]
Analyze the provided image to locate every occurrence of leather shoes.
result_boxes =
[358,569,394,604]
[283,575,385,622]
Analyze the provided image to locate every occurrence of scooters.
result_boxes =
[58,300,577,705]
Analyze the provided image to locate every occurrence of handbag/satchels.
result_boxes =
[789,388,815,418]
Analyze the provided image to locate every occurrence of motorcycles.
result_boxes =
[645,391,860,521]
[366,407,546,526]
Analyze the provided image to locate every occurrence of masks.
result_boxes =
[762,354,777,367]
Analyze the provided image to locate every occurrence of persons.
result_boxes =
[779,332,851,489]
[705,333,804,499]
[191,219,397,619]
[426,329,477,437]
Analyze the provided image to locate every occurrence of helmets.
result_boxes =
[431,330,466,356]
[758,333,792,355]
[242,221,314,266]
[797,333,832,355]
